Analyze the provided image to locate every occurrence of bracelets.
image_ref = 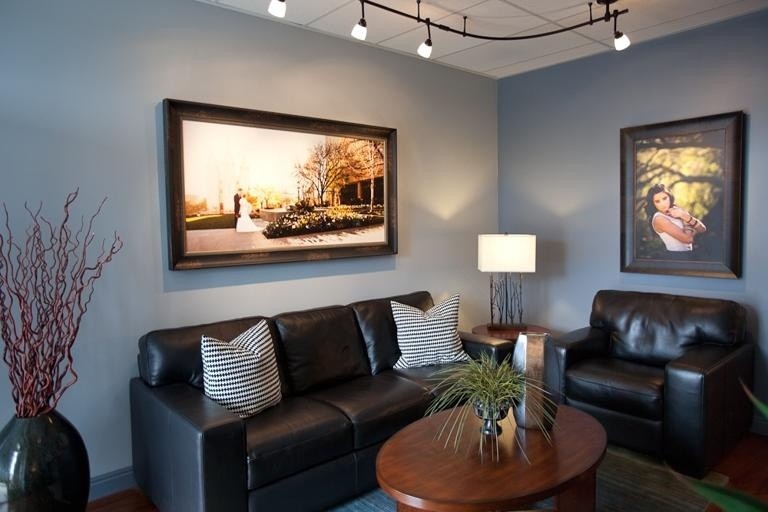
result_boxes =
[685,216,692,223]
[690,220,697,227]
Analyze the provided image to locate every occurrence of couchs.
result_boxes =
[129,288,515,512]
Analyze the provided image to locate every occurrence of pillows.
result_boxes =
[201,318,282,420]
[390,294,475,368]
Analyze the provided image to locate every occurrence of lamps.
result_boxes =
[267,1,632,59]
[476,232,537,332]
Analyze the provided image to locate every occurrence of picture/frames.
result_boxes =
[618,110,744,280]
[162,97,399,271]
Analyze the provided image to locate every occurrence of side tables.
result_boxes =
[471,322,552,345]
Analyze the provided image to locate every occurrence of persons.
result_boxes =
[232,187,243,229]
[237,189,265,233]
[643,183,708,255]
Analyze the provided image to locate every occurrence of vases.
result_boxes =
[0,409,90,512]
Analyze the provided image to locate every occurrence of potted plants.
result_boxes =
[423,348,568,465]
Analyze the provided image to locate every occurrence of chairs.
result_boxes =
[554,289,756,479]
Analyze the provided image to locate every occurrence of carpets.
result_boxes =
[324,443,731,512]
